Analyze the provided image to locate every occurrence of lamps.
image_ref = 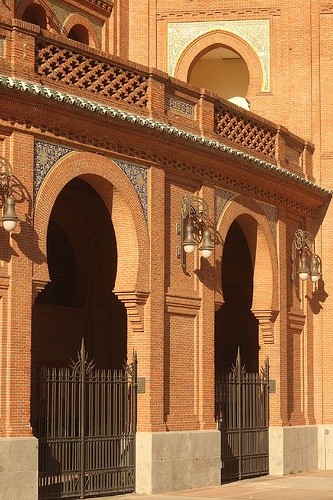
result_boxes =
[179,194,216,276]
[1,156,22,231]
[290,228,322,284]
